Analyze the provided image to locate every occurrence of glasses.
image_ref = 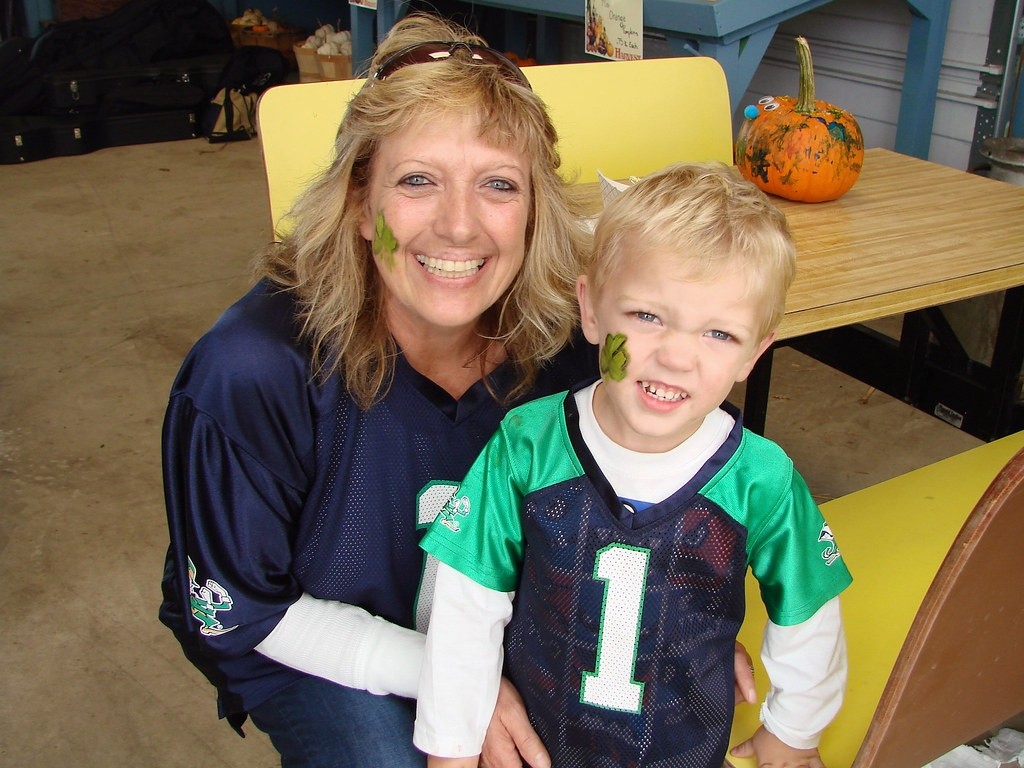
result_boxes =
[369,40,534,96]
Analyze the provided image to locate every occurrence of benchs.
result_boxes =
[726,429,1024,768]
[255,54,736,252]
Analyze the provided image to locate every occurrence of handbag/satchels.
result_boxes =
[202,85,256,144]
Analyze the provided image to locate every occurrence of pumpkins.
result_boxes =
[232,9,536,66]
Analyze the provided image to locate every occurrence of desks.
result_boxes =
[566,146,1024,443]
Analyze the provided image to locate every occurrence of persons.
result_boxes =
[412,163,849,767]
[159,12,604,768]
